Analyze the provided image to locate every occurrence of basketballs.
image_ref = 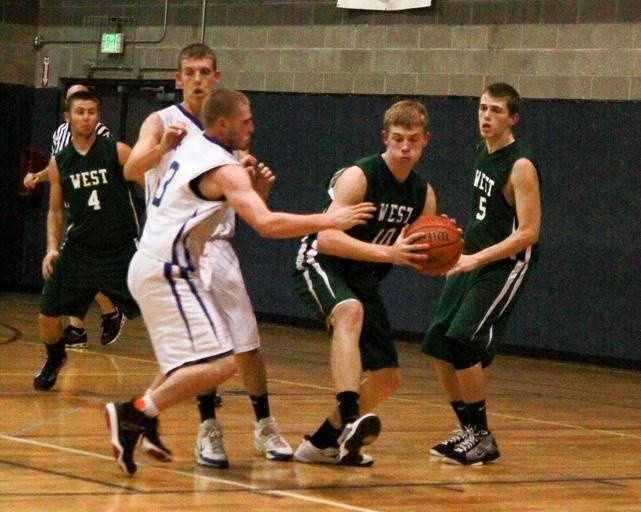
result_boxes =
[405,215,463,276]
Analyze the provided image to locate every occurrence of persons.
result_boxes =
[293,97,467,469]
[103,89,380,479]
[24,84,128,350]
[124,41,296,467]
[32,90,147,393]
[418,82,544,469]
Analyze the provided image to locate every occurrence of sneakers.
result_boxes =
[142,417,173,461]
[34,350,66,389]
[294,435,374,467]
[102,307,126,346]
[194,420,229,468]
[63,325,88,349]
[105,399,148,476]
[430,431,500,466]
[337,414,381,464]
[254,416,294,461]
